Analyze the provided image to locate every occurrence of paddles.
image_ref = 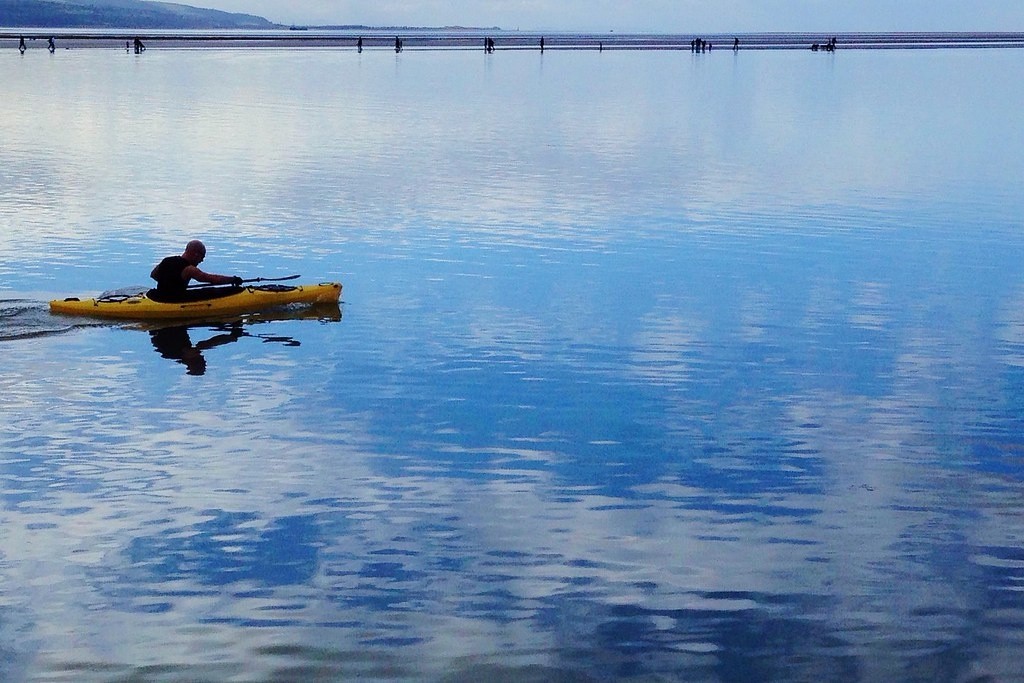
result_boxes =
[98,274,301,299]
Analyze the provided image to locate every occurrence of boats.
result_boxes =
[49,282,342,320]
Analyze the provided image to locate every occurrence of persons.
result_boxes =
[395,36,402,50]
[691,38,712,51]
[126,41,129,48]
[599,41,602,50]
[47,36,55,48]
[134,36,140,48]
[484,37,495,50]
[540,36,544,49]
[812,36,837,50]
[150,240,243,302]
[733,38,739,50]
[356,36,362,50]
[18,36,26,49]
